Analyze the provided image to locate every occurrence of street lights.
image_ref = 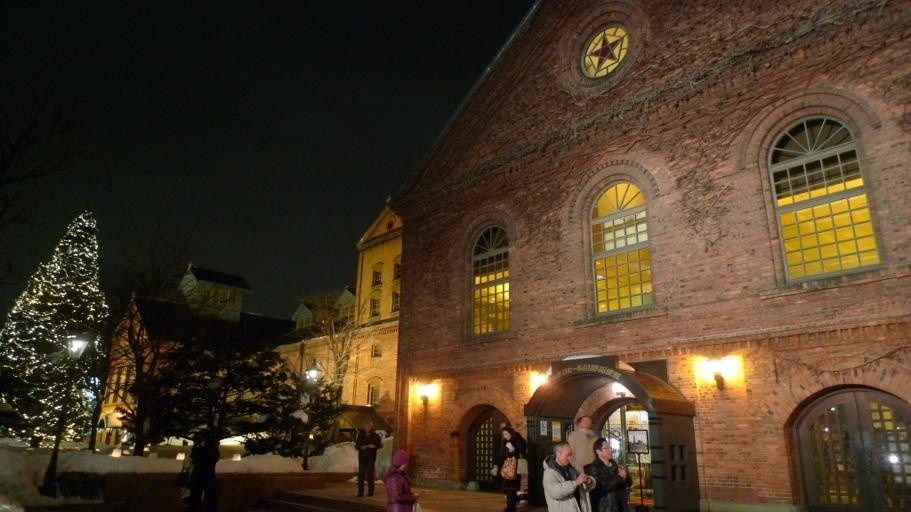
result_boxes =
[302,358,322,469]
[41,316,100,498]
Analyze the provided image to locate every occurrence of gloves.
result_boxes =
[490,465,499,476]
[505,442,515,453]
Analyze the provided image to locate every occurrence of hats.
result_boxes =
[393,450,410,467]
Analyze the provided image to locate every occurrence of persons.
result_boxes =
[355,422,381,497]
[492,427,527,512]
[383,449,426,512]
[583,438,632,512]
[542,441,596,512]
[568,417,599,473]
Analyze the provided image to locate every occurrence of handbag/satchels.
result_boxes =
[175,466,190,488]
[500,456,517,480]
